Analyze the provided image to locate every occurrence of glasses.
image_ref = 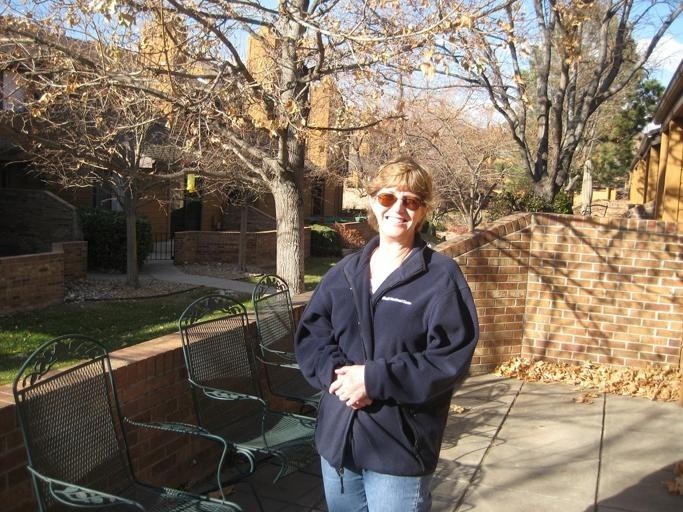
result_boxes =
[374,193,428,212]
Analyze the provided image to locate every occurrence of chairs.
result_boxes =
[10,334,245,511]
[251,275,324,423]
[178,294,323,512]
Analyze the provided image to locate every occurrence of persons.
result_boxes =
[292,156,480,511]
